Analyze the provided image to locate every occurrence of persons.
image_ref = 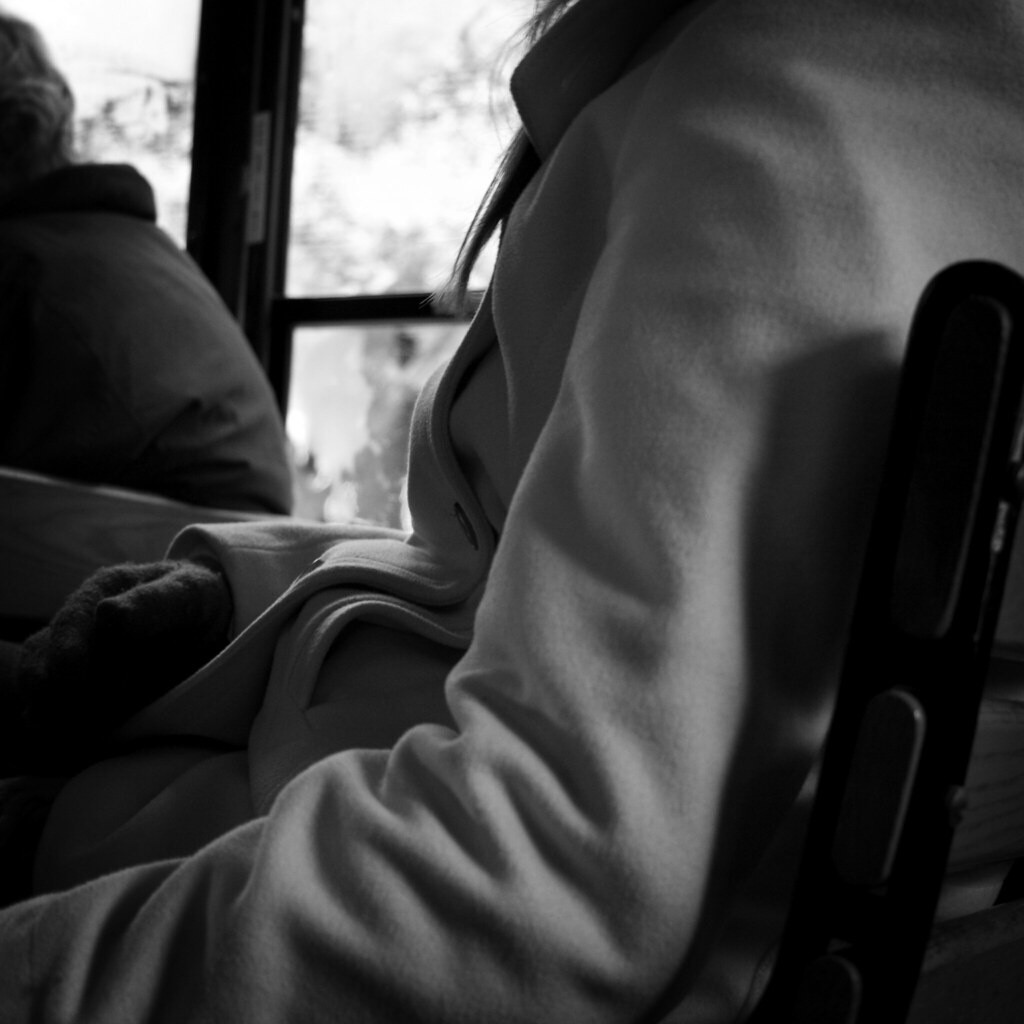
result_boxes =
[0,0,1023,1023]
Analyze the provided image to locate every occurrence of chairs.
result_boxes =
[0,464,264,620]
[749,258,1024,1024]
